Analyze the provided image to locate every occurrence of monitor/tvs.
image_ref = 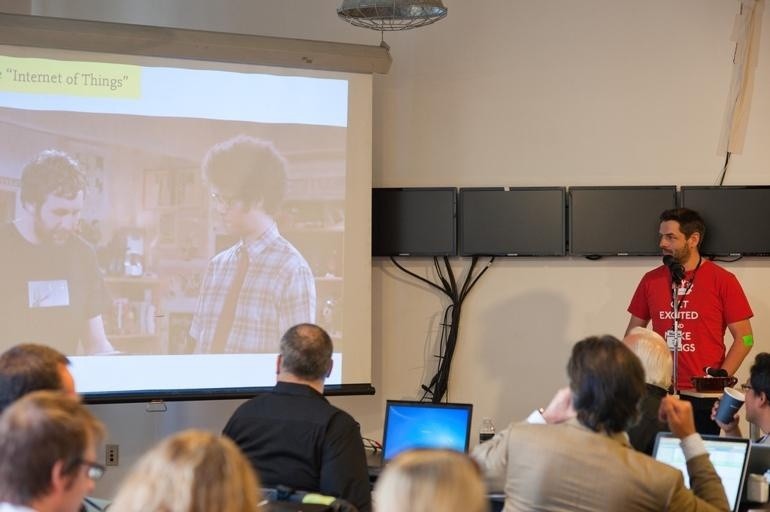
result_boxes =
[372,186,456,257]
[680,185,770,257]
[458,187,565,257]
[567,185,677,257]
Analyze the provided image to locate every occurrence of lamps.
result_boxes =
[337,1,448,32]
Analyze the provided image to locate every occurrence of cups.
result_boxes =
[715,387,745,424]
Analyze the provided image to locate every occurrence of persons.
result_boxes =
[372,447,489,512]
[710,352,770,511]
[0,148,115,356]
[619,327,679,454]
[178,132,317,354]
[624,208,753,435]
[106,429,259,512]
[221,323,370,511]
[0,344,75,412]
[465,334,732,512]
[0,389,106,512]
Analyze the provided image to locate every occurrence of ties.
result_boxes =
[211,257,251,355]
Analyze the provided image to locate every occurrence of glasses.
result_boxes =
[737,382,754,395]
[63,450,106,482]
[210,193,250,206]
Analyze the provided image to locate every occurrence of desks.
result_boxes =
[677,388,760,442]
[362,451,507,512]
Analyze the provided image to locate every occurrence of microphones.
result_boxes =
[662,255,686,282]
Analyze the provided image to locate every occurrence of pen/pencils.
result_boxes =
[84,497,102,512]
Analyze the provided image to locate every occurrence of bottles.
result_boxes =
[479,416,496,444]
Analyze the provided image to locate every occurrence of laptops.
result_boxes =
[652,432,752,511]
[368,399,473,479]
